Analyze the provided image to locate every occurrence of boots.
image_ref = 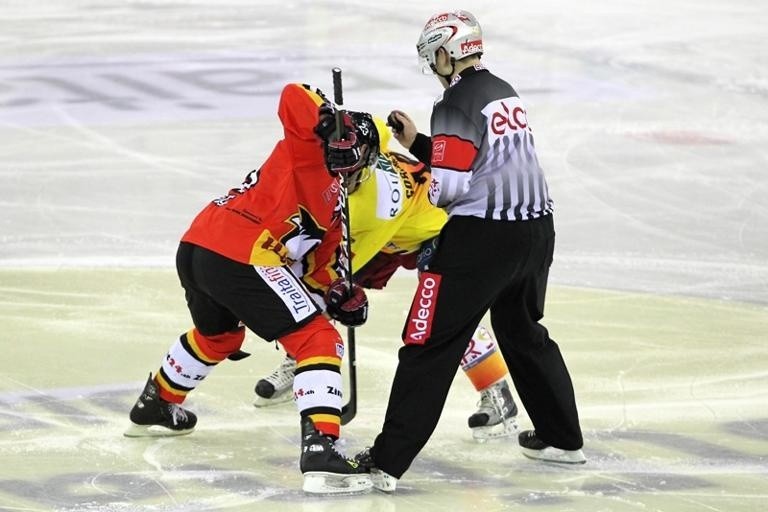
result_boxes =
[129,371,197,432]
[518,430,587,462]
[254,356,298,400]
[299,418,403,479]
[468,379,518,429]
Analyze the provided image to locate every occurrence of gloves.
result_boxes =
[325,278,368,327]
[312,113,361,178]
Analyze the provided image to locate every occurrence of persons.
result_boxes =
[351,10,583,479]
[255,151,518,428]
[129,82,390,474]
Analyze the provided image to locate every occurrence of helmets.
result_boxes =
[347,111,380,182]
[416,9,484,65]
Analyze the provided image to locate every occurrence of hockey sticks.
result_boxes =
[331,67,356,424]
